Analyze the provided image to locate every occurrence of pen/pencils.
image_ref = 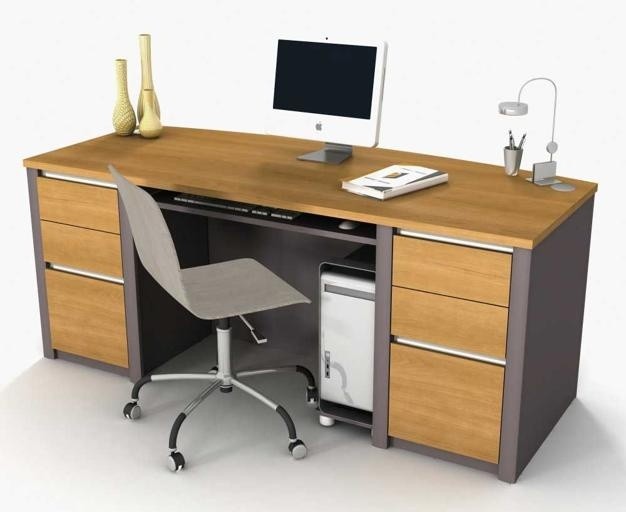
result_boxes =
[509,130,526,150]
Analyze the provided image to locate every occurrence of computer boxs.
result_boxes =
[320,271,376,413]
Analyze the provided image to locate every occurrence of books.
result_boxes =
[340,162,448,200]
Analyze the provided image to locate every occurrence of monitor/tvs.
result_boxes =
[266,34,388,165]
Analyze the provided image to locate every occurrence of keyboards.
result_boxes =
[173,194,301,220]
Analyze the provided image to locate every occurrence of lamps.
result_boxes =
[499,78,561,187]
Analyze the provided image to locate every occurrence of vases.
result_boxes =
[114,59,136,136]
[137,34,160,125]
[139,90,162,139]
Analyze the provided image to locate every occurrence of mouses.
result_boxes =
[338,221,359,230]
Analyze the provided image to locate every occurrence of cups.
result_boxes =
[503,146,524,175]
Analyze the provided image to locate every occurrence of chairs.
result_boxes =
[108,164,320,473]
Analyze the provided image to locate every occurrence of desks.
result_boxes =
[22,127,598,484]
